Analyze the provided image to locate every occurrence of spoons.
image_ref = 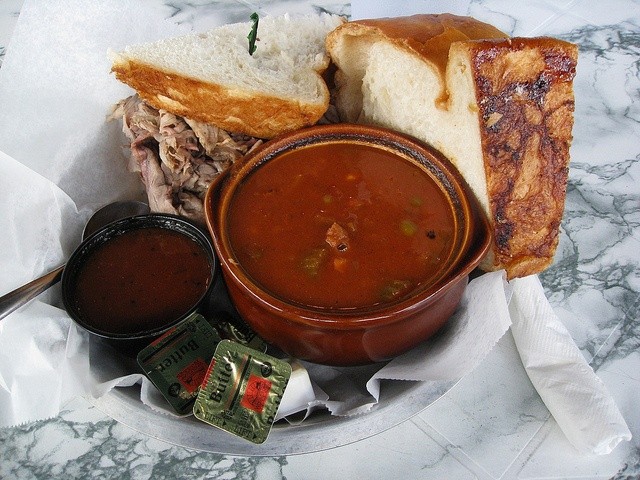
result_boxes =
[0,199,150,321]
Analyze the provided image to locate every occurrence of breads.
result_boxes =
[326,12,578,281]
[109,13,348,140]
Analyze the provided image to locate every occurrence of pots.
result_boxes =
[204,123,494,366]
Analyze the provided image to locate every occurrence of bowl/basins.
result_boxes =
[61,213,219,357]
